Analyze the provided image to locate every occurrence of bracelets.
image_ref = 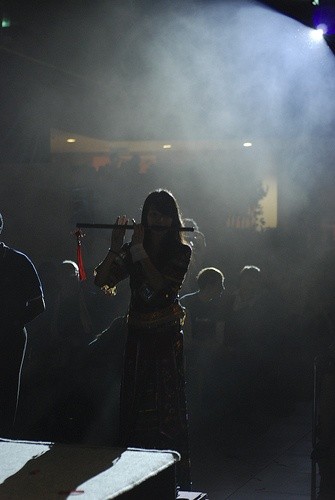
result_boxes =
[109,249,119,256]
[129,243,147,263]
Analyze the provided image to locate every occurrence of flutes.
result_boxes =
[77,223,195,233]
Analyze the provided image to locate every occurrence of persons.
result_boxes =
[94,190,192,491]
[0,216,46,438]
[25,225,335,430]
